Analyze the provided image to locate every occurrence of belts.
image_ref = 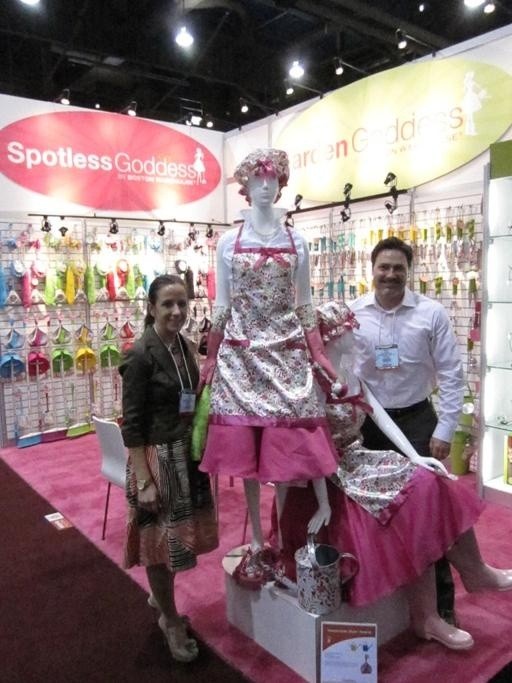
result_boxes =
[384,398,428,420]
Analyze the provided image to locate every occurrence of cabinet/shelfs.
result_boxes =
[475,165,511,506]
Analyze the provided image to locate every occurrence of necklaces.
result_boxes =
[171,344,182,355]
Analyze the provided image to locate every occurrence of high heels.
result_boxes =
[147,592,200,664]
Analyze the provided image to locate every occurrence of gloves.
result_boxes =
[295,303,338,397]
[196,306,231,395]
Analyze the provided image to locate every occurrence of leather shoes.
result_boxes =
[439,609,458,626]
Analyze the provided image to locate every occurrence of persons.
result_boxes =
[308,301,512,654]
[346,237,467,627]
[120,275,218,662]
[190,149,343,576]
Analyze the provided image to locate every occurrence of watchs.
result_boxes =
[135,476,153,491]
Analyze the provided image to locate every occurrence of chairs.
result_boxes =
[89,415,220,542]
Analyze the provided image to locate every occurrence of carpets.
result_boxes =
[2,441,511,683]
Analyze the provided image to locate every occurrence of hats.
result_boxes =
[316,298,360,346]
[234,148,289,206]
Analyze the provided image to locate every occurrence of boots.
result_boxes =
[445,524,512,593]
[406,564,475,651]
